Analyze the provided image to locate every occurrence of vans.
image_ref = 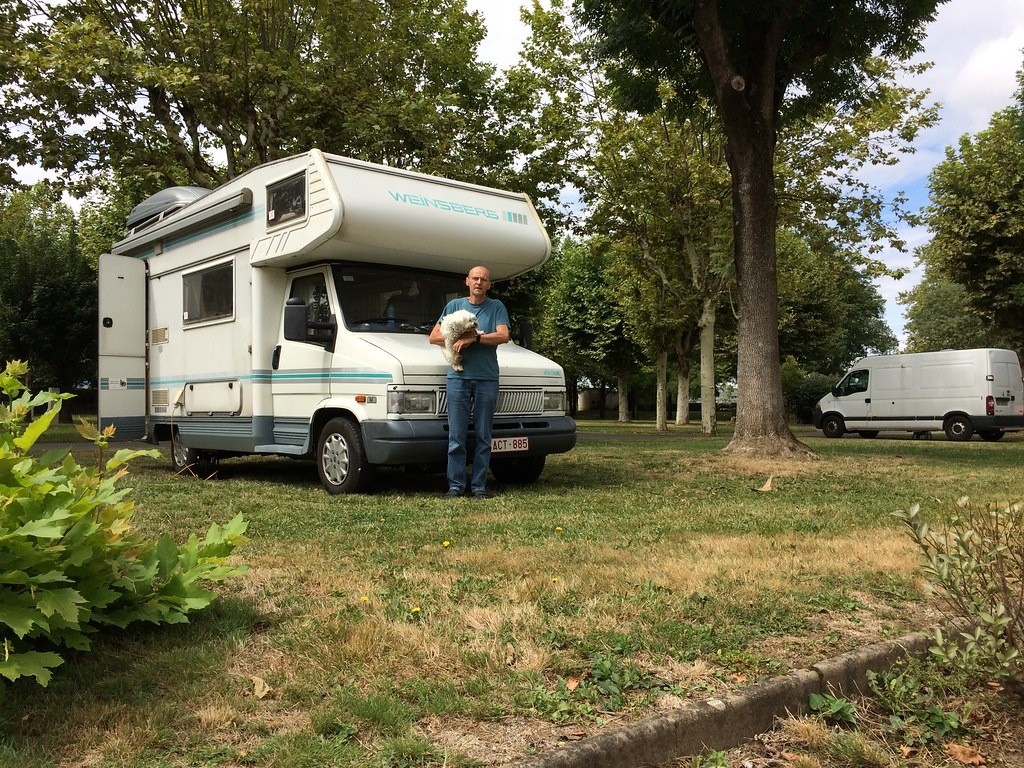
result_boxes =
[96,148,584,498]
[813,347,1024,443]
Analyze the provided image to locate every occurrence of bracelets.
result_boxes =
[477,334,480,342]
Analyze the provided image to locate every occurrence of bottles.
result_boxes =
[386,304,395,328]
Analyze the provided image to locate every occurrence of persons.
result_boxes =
[432,265,511,499]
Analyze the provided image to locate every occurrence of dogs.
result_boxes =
[439,308,487,372]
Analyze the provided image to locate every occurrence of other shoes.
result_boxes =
[443,488,458,498]
[474,491,489,500]
